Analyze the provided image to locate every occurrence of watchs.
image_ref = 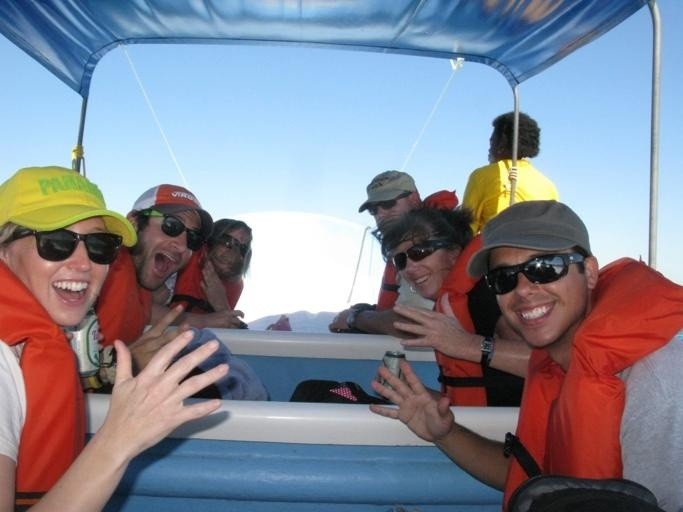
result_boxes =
[346,308,363,329]
[480,336,494,366]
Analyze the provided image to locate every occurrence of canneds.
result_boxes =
[65,308,101,378]
[379,348,404,390]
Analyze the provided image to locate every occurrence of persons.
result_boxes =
[370,199,683,511]
[381,201,526,406]
[0,166,229,512]
[84,184,218,385]
[149,220,252,328]
[462,109,559,237]
[329,170,459,339]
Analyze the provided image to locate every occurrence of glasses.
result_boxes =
[143,209,207,252]
[216,233,249,256]
[392,239,453,272]
[1,227,124,266]
[483,252,587,296]
[366,192,412,217]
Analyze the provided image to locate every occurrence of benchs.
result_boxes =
[80,389,521,511]
[134,320,445,401]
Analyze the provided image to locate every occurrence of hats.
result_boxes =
[466,199,593,277]
[131,184,213,242]
[0,165,139,248]
[358,169,417,214]
